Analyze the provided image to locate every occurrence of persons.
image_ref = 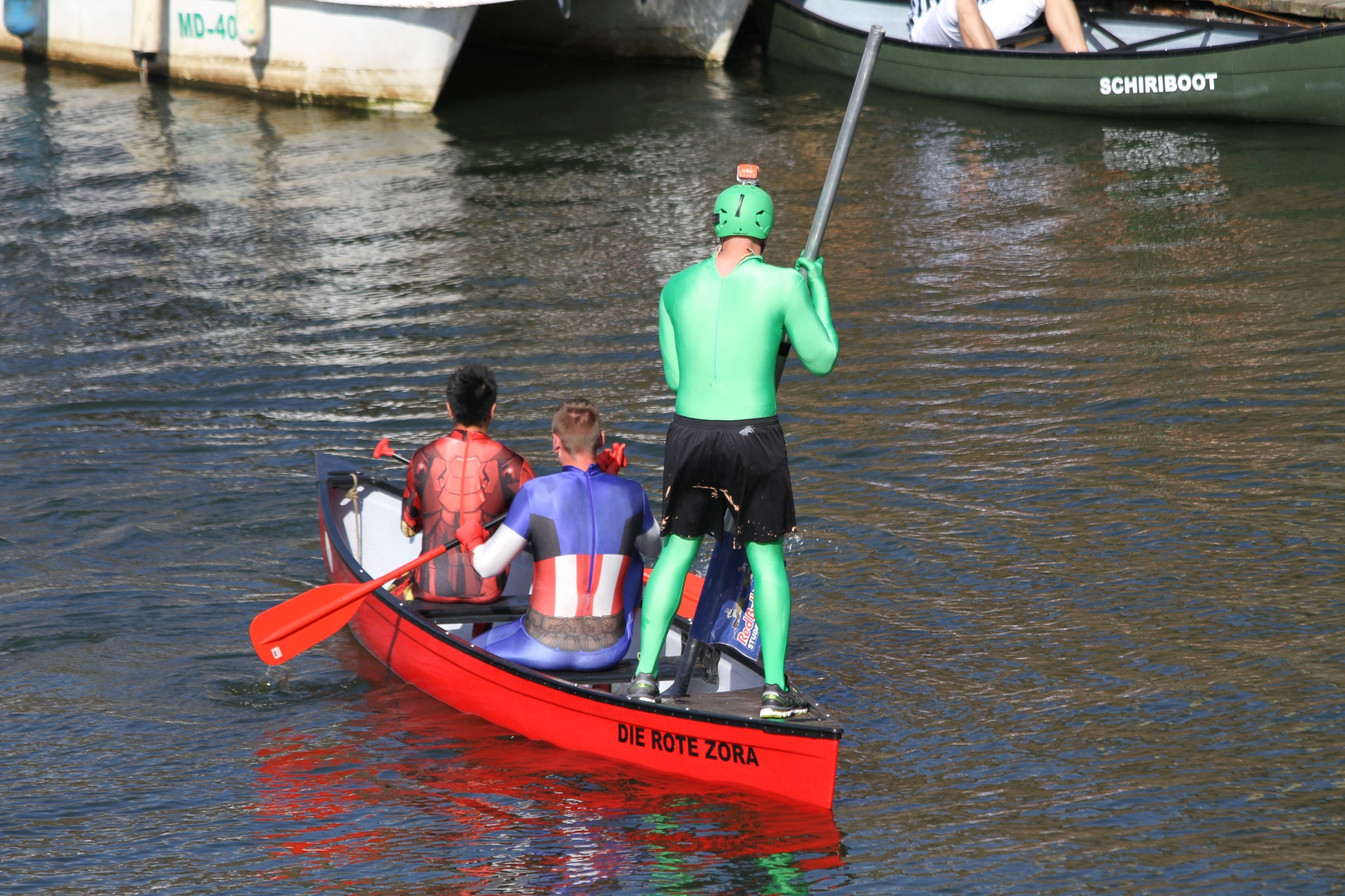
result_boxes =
[908,2,1089,52]
[626,185,839,718]
[455,400,662,671]
[387,364,536,650]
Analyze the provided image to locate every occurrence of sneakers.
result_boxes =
[760,674,810,718]
[626,672,662,703]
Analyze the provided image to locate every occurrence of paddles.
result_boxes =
[248,510,509,668]
[369,436,706,622]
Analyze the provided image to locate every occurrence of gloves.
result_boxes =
[455,518,489,551]
[597,443,628,476]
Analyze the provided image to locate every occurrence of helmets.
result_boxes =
[712,185,774,239]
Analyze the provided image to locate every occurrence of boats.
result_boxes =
[752,1,1344,129]
[314,448,845,808]
[1,0,750,109]
